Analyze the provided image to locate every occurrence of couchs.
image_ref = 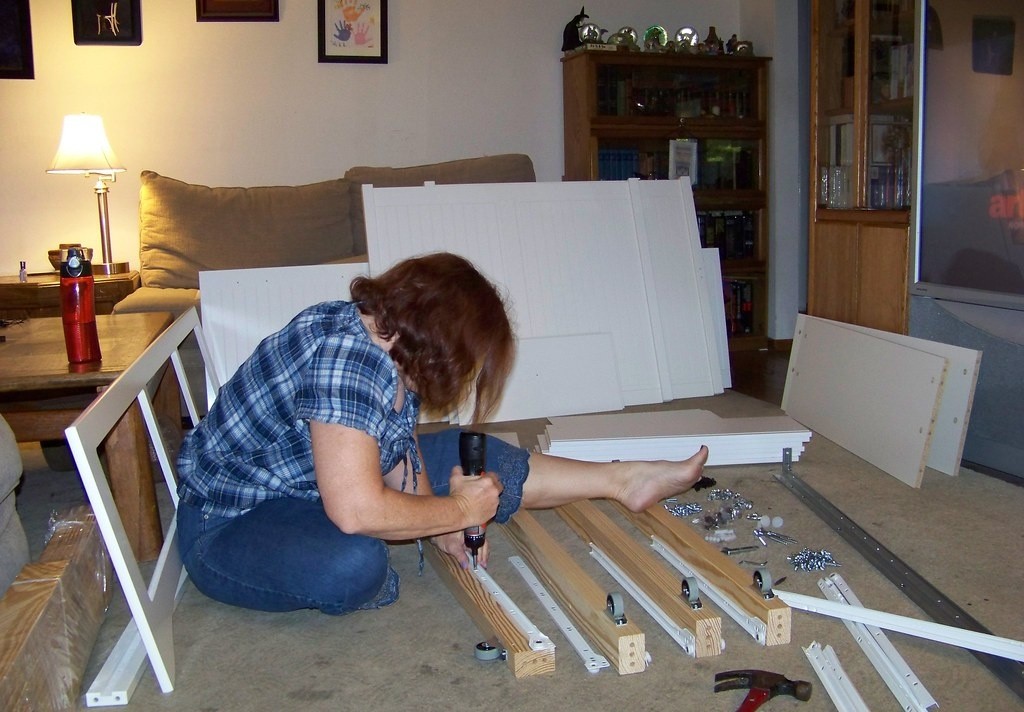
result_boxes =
[112,153,537,429]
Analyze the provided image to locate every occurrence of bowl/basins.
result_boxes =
[48,244,86,269]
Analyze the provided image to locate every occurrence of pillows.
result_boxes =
[139,170,356,288]
[345,153,537,255]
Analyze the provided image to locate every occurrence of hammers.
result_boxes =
[713,669,813,712]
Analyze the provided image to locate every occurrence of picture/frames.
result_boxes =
[973,15,1015,76]
[71,0,143,46]
[317,0,389,65]
[195,0,280,23]
[0,0,35,80]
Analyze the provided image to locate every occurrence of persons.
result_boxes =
[174,252,709,618]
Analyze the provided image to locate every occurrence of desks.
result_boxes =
[0,313,181,561]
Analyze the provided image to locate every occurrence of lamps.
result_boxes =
[45,114,130,275]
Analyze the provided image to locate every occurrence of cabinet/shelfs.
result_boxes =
[560,51,774,351]
[807,0,915,335]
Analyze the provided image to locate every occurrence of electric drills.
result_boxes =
[459,431,487,572]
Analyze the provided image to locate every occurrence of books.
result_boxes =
[595,64,758,120]
[889,43,913,100]
[819,165,853,210]
[598,147,668,180]
[724,255,748,272]
[870,0,914,36]
[696,210,754,256]
[870,147,912,209]
[829,122,853,167]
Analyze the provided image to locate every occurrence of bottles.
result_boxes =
[60,249,102,362]
[705,26,719,46]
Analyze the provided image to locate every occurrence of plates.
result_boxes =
[619,28,637,43]
[675,27,698,46]
[643,25,667,46]
[580,24,602,42]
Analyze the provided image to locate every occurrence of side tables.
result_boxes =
[0,270,141,331]
